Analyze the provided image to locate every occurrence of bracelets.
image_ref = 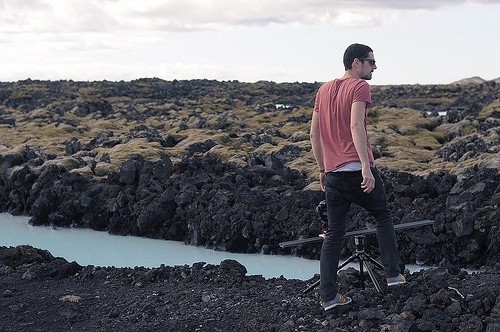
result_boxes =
[318,169,325,173]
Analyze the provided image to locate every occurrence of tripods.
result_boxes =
[303,233,385,298]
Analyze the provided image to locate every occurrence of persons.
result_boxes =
[310,43,406,312]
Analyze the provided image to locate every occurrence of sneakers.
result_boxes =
[322,293,352,311]
[387,273,406,286]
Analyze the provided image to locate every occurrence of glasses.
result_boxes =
[358,58,375,65]
